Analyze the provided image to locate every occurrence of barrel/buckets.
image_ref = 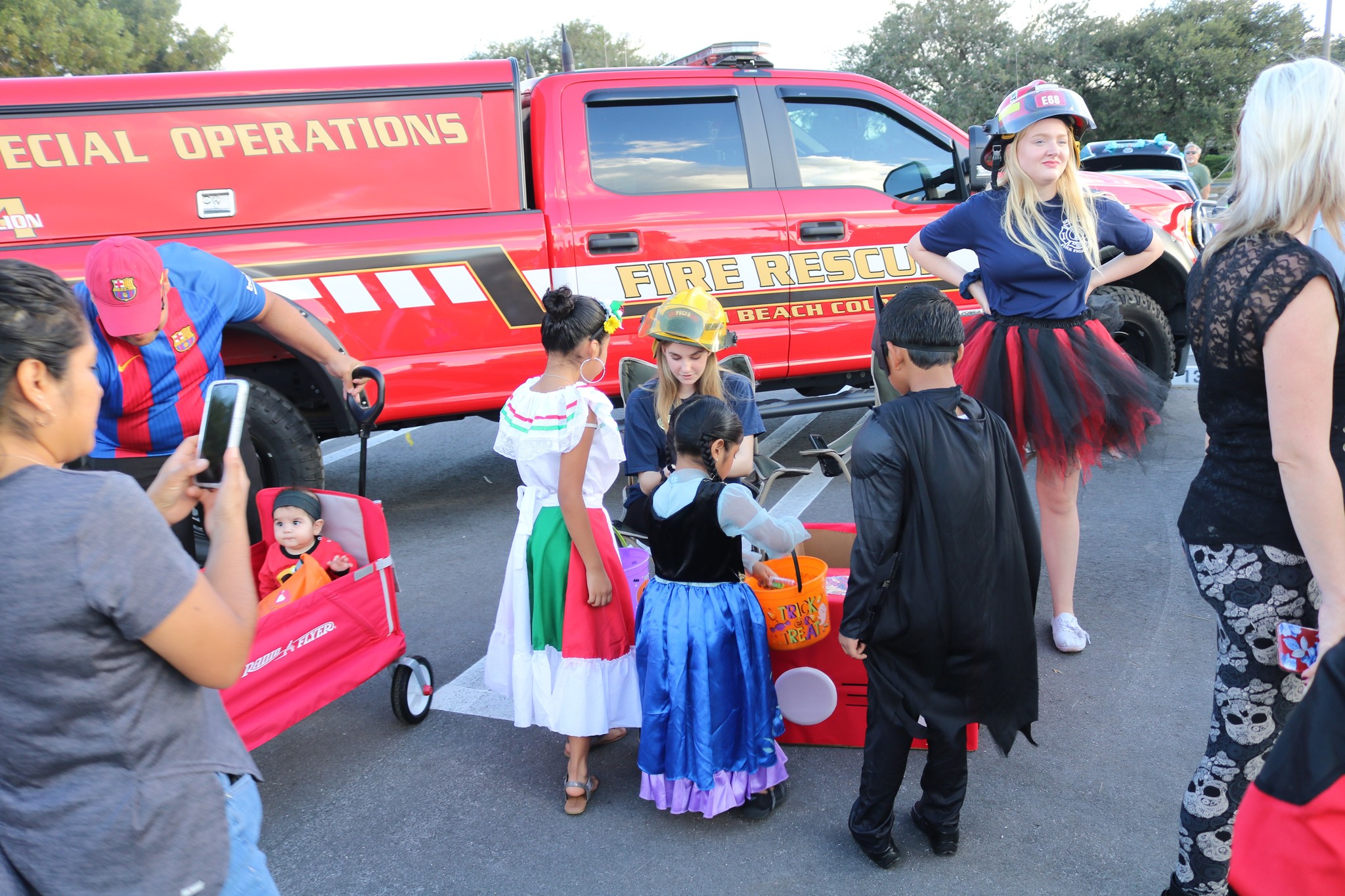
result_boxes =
[613,525,650,620]
[744,547,831,650]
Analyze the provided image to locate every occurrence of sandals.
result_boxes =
[563,773,595,814]
[738,779,786,818]
[565,726,628,758]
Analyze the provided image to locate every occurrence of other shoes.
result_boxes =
[1052,612,1090,652]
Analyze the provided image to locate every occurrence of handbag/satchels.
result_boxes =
[258,554,331,619]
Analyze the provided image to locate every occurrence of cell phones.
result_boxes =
[192,378,250,490]
[809,434,844,476]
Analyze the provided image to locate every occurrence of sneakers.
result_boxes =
[853,830,901,870]
[912,798,960,856]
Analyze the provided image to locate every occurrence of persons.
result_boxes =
[1160,59,1345,896]
[643,393,793,820]
[497,285,630,816]
[620,289,759,543]
[1182,143,1212,200]
[1,258,282,896]
[837,285,1019,869]
[258,486,361,600]
[68,236,372,559]
[905,76,1167,651]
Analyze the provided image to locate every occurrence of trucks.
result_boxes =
[1,20,1218,550]
[1075,132,1208,217]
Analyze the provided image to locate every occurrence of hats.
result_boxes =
[85,234,163,339]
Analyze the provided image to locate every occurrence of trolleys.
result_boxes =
[199,365,435,755]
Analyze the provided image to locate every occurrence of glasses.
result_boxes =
[1185,151,1197,154]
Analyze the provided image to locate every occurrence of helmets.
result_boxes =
[648,286,725,347]
[980,78,1086,171]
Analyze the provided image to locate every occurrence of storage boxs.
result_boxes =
[761,523,979,752]
